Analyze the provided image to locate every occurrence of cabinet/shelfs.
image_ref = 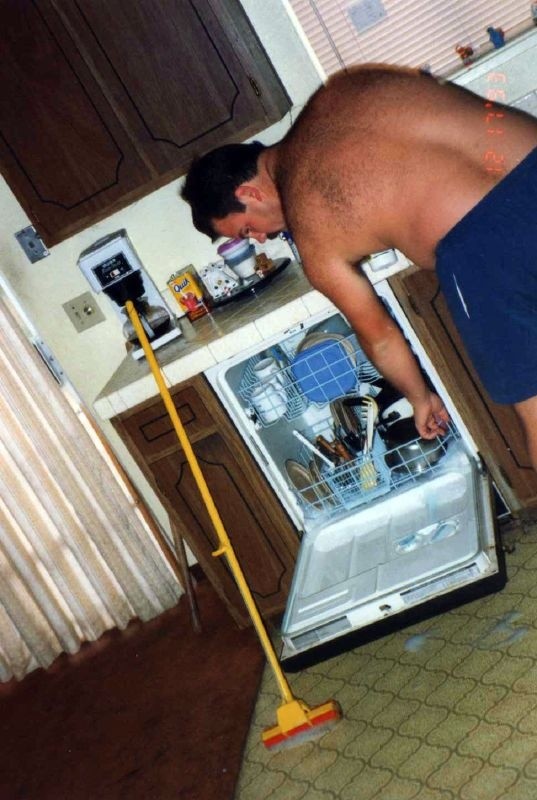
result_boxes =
[386,262,537,519]
[0,0,293,249]
[109,371,302,631]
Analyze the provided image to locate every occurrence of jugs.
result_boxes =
[122,297,170,344]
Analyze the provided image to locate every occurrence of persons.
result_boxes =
[180,62,537,471]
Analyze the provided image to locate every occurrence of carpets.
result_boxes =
[0,576,265,800]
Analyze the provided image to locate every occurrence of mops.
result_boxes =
[125,300,345,754]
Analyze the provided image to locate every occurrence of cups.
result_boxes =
[250,356,289,424]
[212,234,258,279]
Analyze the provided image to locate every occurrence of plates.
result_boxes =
[286,458,337,510]
[289,314,379,403]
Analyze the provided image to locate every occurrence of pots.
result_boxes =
[385,417,448,473]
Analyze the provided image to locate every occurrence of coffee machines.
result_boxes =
[77,229,183,362]
